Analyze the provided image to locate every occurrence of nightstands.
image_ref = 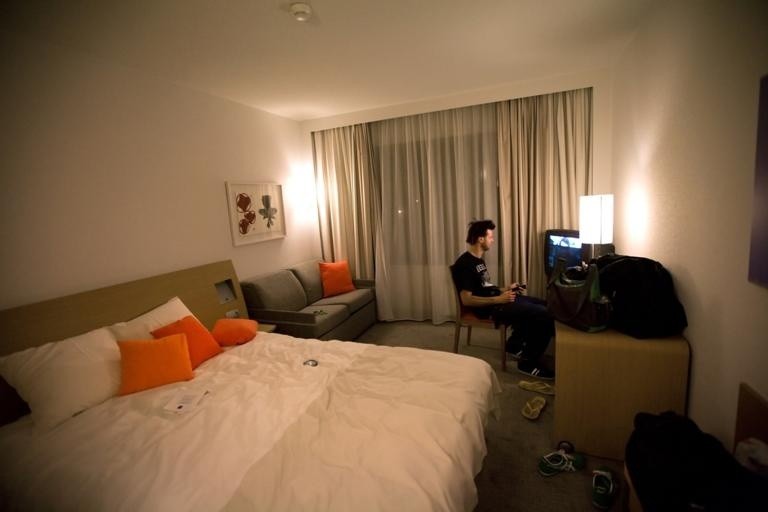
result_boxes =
[257,323,276,332]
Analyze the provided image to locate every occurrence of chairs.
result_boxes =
[449,264,512,371]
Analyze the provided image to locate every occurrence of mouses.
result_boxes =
[304,359,317,367]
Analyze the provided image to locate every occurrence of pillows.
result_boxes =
[1,297,257,442]
[318,258,356,297]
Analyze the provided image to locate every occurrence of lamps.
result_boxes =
[579,194,615,264]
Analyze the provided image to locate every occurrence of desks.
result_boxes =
[555,320,689,461]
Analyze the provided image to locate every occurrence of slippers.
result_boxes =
[521,397,545,419]
[517,380,554,395]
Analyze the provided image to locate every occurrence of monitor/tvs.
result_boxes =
[544,229,615,275]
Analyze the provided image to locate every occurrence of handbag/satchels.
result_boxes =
[554,255,686,340]
[624,410,748,512]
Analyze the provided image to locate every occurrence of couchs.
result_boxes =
[240,258,377,340]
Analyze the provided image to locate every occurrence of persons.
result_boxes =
[560,238,571,247]
[453,220,554,381]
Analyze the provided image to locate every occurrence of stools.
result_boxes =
[624,461,642,512]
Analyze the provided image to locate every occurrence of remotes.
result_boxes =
[512,285,526,292]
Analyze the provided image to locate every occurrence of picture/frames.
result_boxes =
[225,183,287,246]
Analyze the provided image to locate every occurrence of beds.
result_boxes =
[0,260,502,512]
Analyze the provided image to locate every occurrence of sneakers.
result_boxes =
[537,452,585,477]
[517,365,554,380]
[591,463,613,511]
